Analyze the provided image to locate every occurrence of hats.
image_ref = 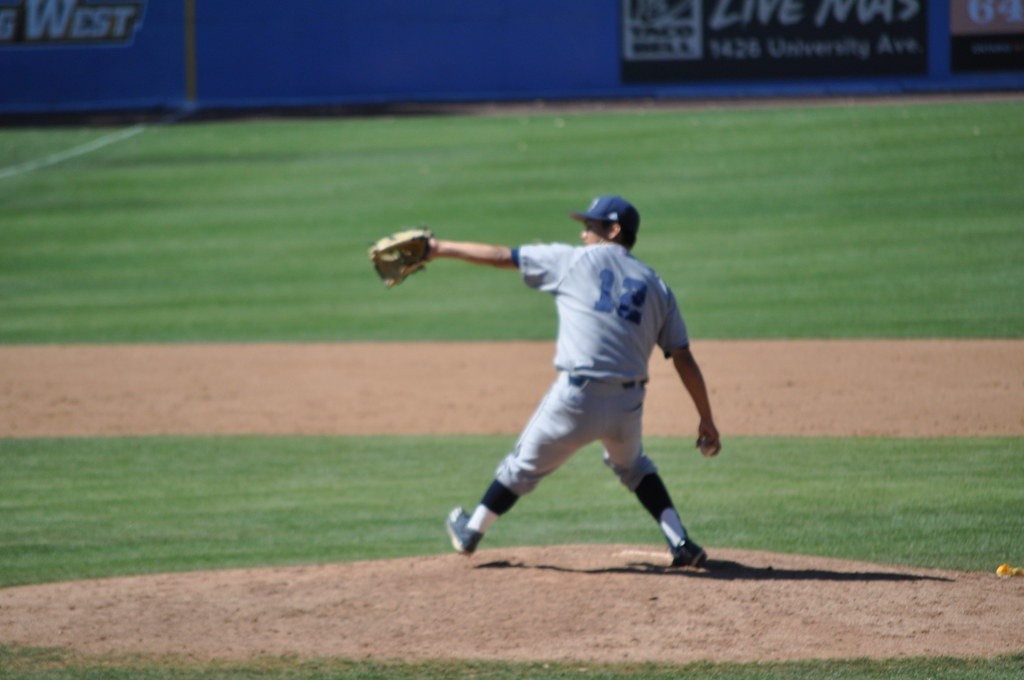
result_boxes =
[570,194,639,234]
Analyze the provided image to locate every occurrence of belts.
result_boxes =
[568,374,645,389]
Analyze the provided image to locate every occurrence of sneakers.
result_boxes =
[670,535,707,567]
[447,507,483,555]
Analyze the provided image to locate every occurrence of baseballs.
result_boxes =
[699,439,717,458]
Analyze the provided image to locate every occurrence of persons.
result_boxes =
[367,195,723,567]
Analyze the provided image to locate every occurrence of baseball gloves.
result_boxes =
[366,227,435,290]
